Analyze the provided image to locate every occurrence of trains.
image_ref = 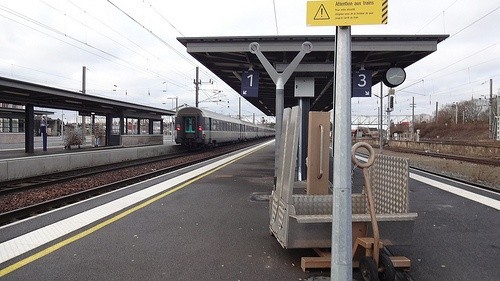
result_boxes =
[174,105,275,147]
